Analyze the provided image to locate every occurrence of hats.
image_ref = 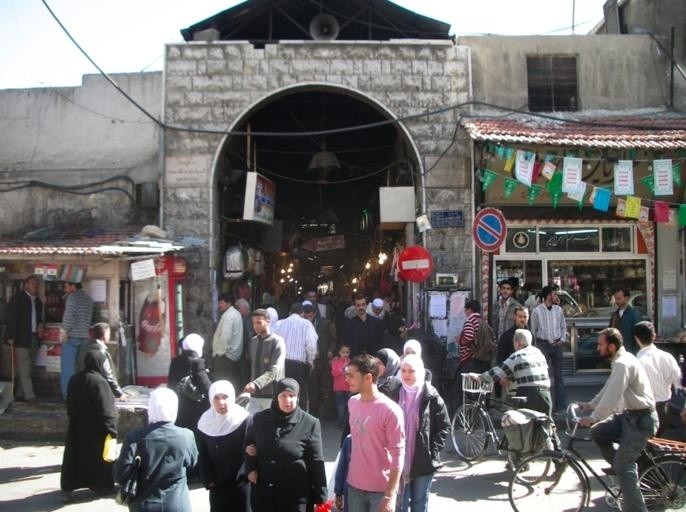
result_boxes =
[373,298,384,307]
[302,300,312,306]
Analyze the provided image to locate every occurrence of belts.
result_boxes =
[656,401,669,406]
[285,359,305,366]
[467,358,481,361]
[251,483,282,487]
[32,332,38,335]
[625,407,657,415]
[537,338,561,345]
[517,386,550,391]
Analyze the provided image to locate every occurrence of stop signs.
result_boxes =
[398,246,433,284]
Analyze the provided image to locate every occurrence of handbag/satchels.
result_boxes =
[117,430,142,506]
[175,358,208,406]
[234,415,250,489]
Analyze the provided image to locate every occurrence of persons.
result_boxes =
[375,347,401,388]
[400,339,432,384]
[496,306,536,364]
[49,280,94,404]
[60,349,121,503]
[381,318,406,345]
[632,320,683,438]
[235,298,255,386]
[367,289,380,305]
[167,333,212,431]
[330,344,351,431]
[75,322,128,403]
[608,287,641,356]
[194,379,257,512]
[334,433,352,512]
[488,280,522,409]
[448,299,498,431]
[343,293,380,359]
[345,354,406,512]
[212,294,244,381]
[266,307,278,333]
[306,290,327,317]
[378,354,452,512]
[112,387,199,512]
[530,285,569,414]
[471,328,568,479]
[5,275,44,405]
[243,308,286,414]
[302,304,337,417]
[277,303,319,379]
[575,328,660,512]
[366,298,390,322]
[241,378,328,512]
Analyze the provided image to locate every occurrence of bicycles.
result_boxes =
[508,403,686,512]
[451,373,553,485]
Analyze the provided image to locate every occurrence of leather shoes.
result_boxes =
[601,468,618,475]
[28,399,40,405]
[16,397,26,402]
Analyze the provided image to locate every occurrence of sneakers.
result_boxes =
[62,490,75,502]
[93,485,120,496]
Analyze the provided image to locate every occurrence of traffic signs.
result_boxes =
[473,207,507,251]
